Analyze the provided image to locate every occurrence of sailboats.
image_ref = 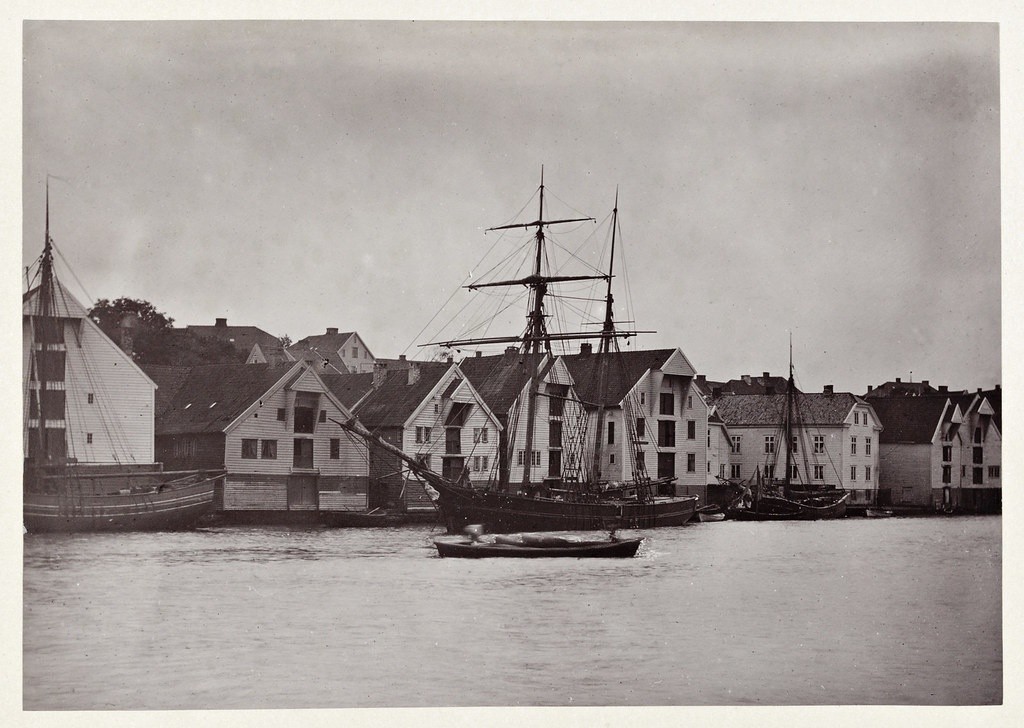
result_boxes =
[726,332,852,519]
[326,161,697,527]
[23,176,227,529]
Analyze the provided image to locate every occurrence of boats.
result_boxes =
[434,524,644,558]
